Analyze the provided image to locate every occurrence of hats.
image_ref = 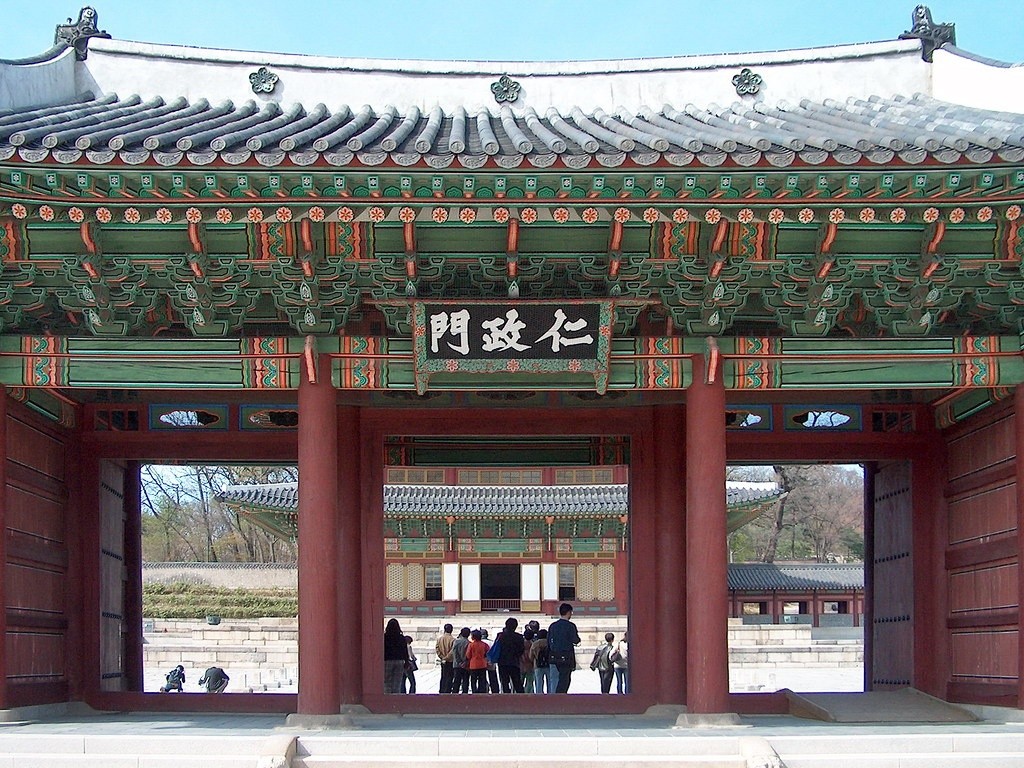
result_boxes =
[480,629,488,635]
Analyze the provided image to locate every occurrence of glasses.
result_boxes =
[570,611,573,615]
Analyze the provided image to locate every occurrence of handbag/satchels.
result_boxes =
[548,651,567,664]
[486,636,502,663]
[405,660,418,672]
[611,642,622,663]
[590,646,608,671]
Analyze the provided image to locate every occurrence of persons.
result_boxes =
[384,603,629,695]
[199,667,229,693]
[159,664,185,692]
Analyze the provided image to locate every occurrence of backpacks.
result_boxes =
[534,645,550,668]
